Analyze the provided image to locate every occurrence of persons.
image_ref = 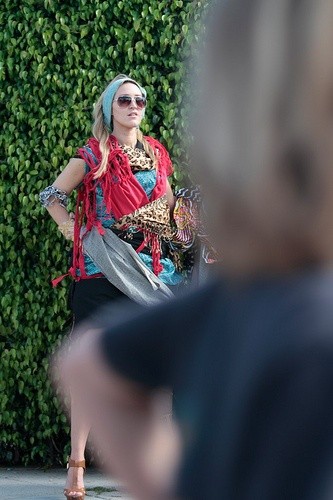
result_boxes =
[37,74,217,500]
[52,1,333,499]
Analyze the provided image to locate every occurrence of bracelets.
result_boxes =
[58,219,75,241]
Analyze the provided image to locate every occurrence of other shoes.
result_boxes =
[62,457,85,500]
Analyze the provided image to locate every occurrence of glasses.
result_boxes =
[111,96,146,110]
